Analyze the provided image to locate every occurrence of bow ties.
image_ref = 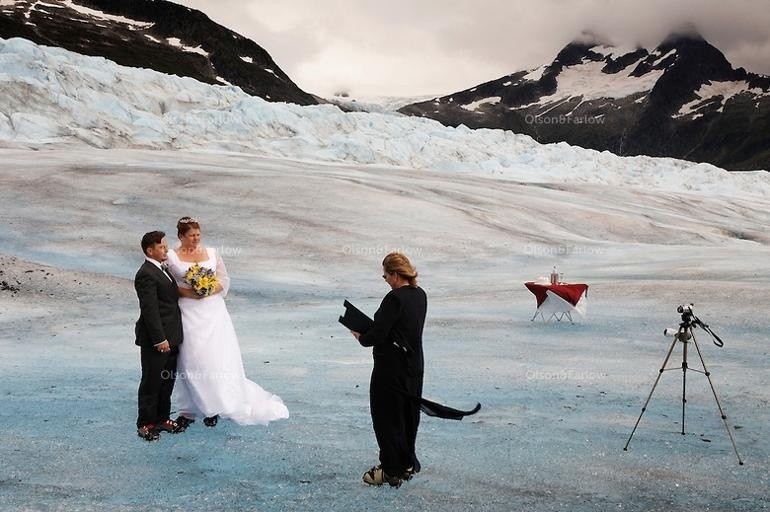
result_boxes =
[161,263,169,270]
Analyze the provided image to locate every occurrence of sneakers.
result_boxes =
[156,419,185,433]
[137,424,161,439]
[203,416,218,427]
[175,416,192,427]
[363,464,415,486]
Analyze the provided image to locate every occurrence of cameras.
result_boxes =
[677,304,695,314]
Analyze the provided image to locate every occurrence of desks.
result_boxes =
[526,283,588,324]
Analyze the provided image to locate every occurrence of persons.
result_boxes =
[162,216,291,429]
[133,230,184,441]
[353,253,427,487]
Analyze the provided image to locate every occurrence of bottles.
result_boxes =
[551,265,558,285]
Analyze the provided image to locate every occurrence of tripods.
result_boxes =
[623,315,746,465]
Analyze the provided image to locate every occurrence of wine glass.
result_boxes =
[559,272,565,285]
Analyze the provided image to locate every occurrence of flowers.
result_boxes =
[183,262,218,297]
